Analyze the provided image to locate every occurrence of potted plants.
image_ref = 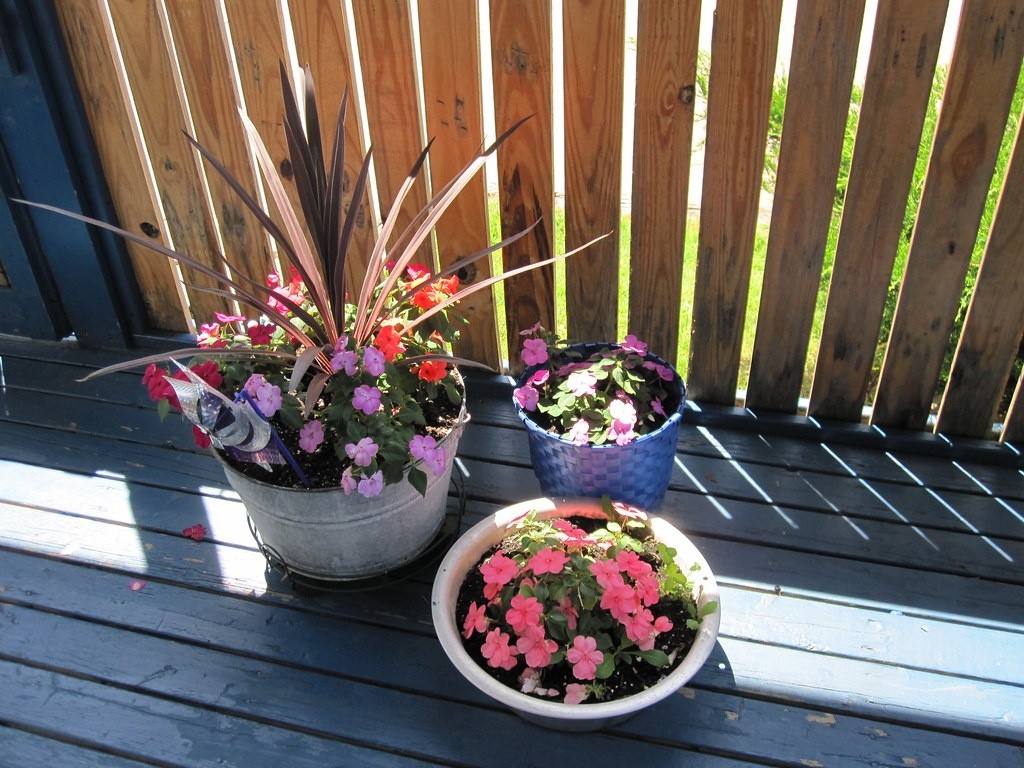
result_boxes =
[10,59,613,581]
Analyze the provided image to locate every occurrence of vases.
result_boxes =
[513,342,685,514]
[431,495,721,732]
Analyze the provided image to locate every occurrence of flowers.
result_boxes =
[513,321,674,446]
[142,260,459,498]
[463,495,673,704]
